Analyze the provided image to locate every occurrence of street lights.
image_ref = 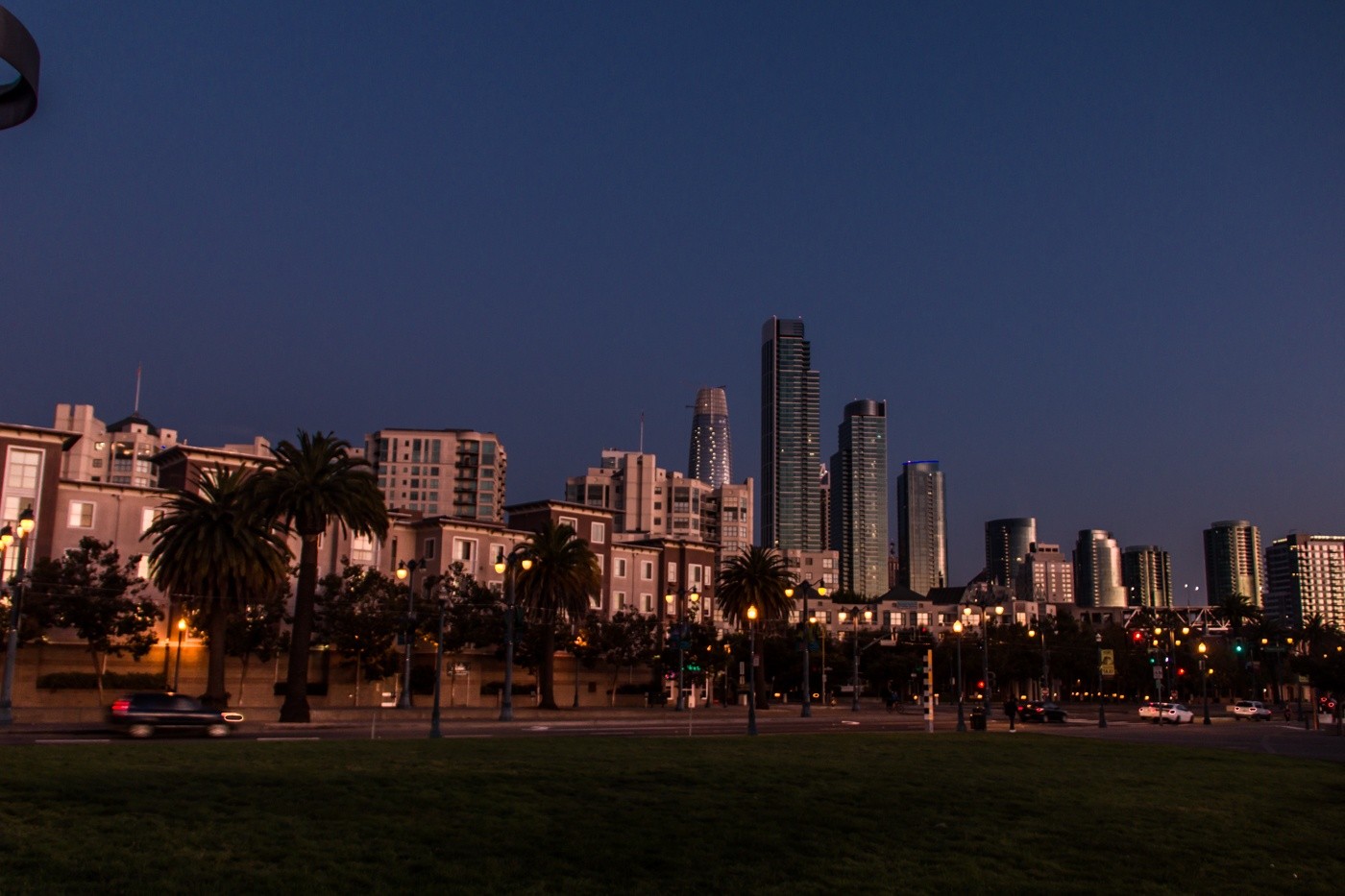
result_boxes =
[494,545,534,723]
[664,584,701,712]
[394,556,428,710]
[964,599,1005,709]
[784,576,827,718]
[838,605,874,712]
[0,502,37,729]
[952,620,967,731]
[1153,639,1182,698]
[744,601,760,736]
[1154,625,1191,691]
[172,618,187,692]
[1198,642,1211,725]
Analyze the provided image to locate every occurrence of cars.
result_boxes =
[102,690,245,740]
[1153,703,1196,726]
[1138,701,1171,723]
[1015,700,1069,724]
[1233,700,1272,722]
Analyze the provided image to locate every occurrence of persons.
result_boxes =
[1006,695,1021,732]
[1283,697,1340,730]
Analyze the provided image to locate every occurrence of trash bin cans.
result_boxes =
[970,706,986,731]
